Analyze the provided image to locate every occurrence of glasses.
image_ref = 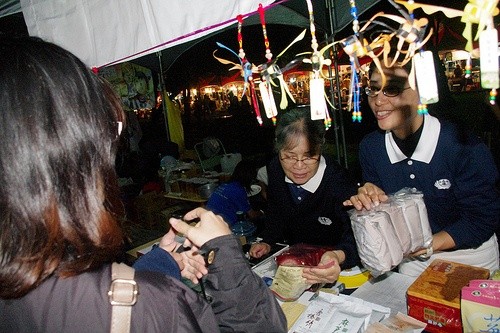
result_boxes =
[279,151,320,165]
[365,84,411,97]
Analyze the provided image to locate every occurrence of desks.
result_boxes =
[125,192,416,330]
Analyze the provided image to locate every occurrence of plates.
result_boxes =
[246,185,261,196]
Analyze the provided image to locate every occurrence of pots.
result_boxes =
[198,182,217,198]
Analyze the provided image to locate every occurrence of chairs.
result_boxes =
[194,138,226,172]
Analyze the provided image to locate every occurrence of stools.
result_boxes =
[134,201,168,230]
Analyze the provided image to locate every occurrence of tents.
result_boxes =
[1,0,381,170]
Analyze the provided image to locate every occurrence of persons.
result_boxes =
[256,107,360,285]
[342,50,500,278]
[256,156,280,202]
[206,160,256,228]
[454,64,463,78]
[1,36,287,333]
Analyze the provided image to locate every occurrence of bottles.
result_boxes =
[234,211,256,252]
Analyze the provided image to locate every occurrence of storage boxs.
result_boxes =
[406,259,490,333]
